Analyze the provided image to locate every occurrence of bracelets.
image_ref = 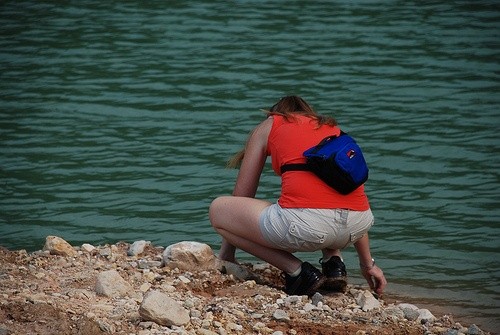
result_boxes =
[360,258,375,271]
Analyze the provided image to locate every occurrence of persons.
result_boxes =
[208,96,388,298]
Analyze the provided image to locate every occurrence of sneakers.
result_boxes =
[283,260,326,296]
[320,256,348,287]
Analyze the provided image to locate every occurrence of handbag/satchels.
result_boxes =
[303,133,369,196]
